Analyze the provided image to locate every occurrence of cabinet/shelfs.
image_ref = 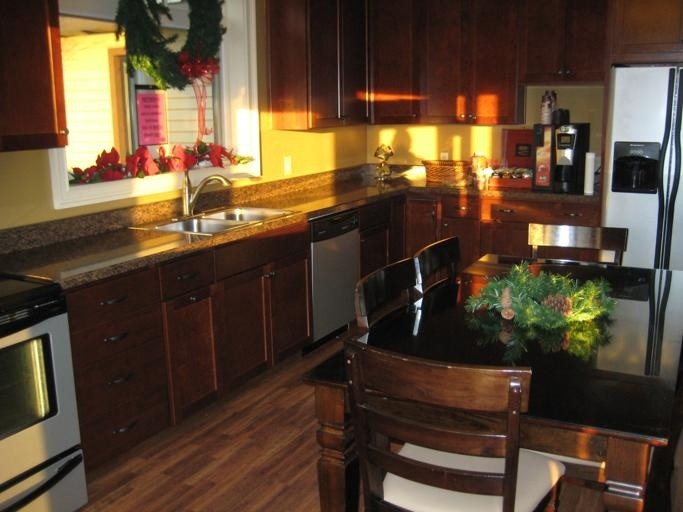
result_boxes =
[527,0,609,80]
[0,0,69,151]
[426,0,516,117]
[266,0,366,131]
[374,0,415,117]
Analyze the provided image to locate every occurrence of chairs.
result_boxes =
[355,257,423,329]
[414,235,460,294]
[343,338,566,512]
[528,222,628,266]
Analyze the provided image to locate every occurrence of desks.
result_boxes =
[311,253,683,512]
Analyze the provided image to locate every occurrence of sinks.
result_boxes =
[128,217,252,237]
[197,205,303,222]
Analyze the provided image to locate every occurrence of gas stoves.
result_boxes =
[0,272,60,309]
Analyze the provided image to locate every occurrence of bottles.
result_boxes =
[540,90,571,125]
[492,166,532,178]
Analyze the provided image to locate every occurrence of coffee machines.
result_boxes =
[531,122,591,192]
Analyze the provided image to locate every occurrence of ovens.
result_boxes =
[0,291,89,511]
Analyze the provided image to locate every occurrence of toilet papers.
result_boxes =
[584,152,595,195]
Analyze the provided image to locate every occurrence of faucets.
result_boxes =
[181,169,232,216]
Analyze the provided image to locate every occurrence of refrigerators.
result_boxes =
[597,63,682,271]
[594,266,683,382]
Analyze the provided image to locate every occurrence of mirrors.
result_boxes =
[57,13,230,205]
